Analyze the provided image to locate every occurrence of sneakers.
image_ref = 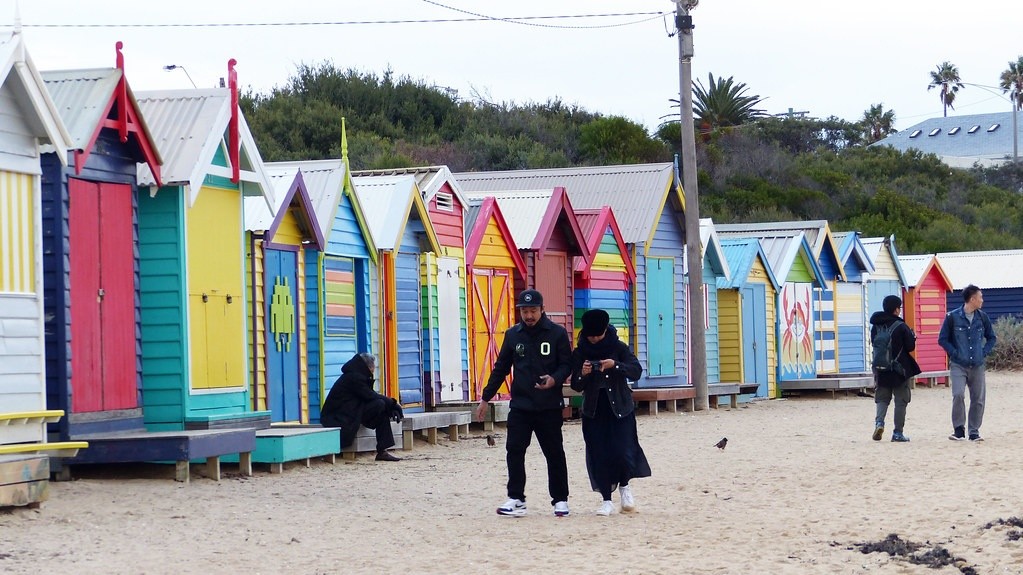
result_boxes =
[619,485,634,511]
[497,498,526,517]
[554,500,569,515]
[597,500,614,515]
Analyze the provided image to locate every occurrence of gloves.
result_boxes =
[392,403,405,425]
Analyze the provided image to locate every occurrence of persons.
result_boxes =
[870,296,922,442]
[938,284,997,440]
[476,290,572,517]
[570,309,651,515]
[319,353,404,461]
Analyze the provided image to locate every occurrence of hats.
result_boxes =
[516,290,543,307]
[581,308,610,337]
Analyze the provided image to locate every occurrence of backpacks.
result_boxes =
[872,320,905,385]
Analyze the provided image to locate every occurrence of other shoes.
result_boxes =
[375,452,399,461]
[968,435,982,441]
[892,435,909,442]
[949,433,966,441]
[873,425,884,441]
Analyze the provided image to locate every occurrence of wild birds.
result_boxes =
[485,435,496,449]
[713,438,729,450]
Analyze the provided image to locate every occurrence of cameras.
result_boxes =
[590,360,601,371]
